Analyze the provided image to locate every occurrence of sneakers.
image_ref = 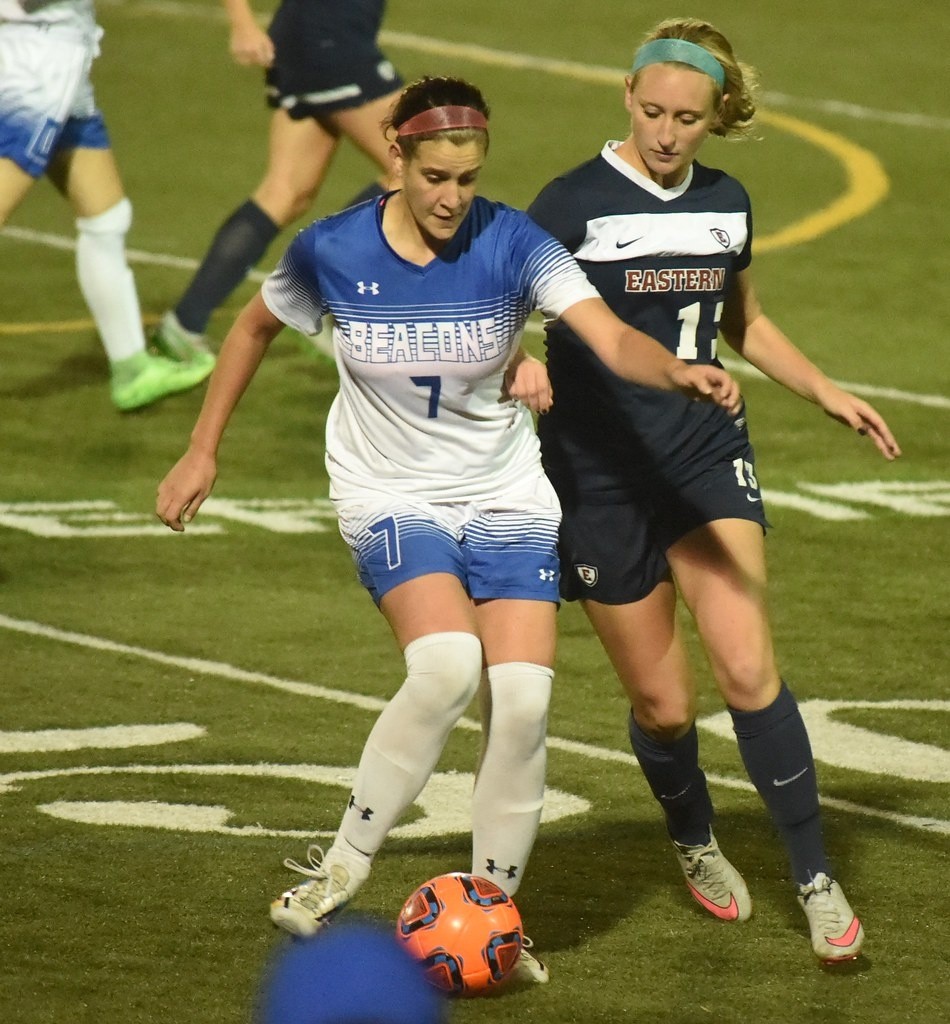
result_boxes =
[671,823,750,922]
[797,870,865,967]
[510,934,549,986]
[108,348,215,411]
[148,309,215,364]
[268,844,352,941]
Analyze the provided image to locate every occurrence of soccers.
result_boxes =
[395,872,523,998]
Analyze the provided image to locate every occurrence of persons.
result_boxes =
[151,2,414,365]
[156,76,742,989]
[501,17,905,963]
[1,0,219,412]
